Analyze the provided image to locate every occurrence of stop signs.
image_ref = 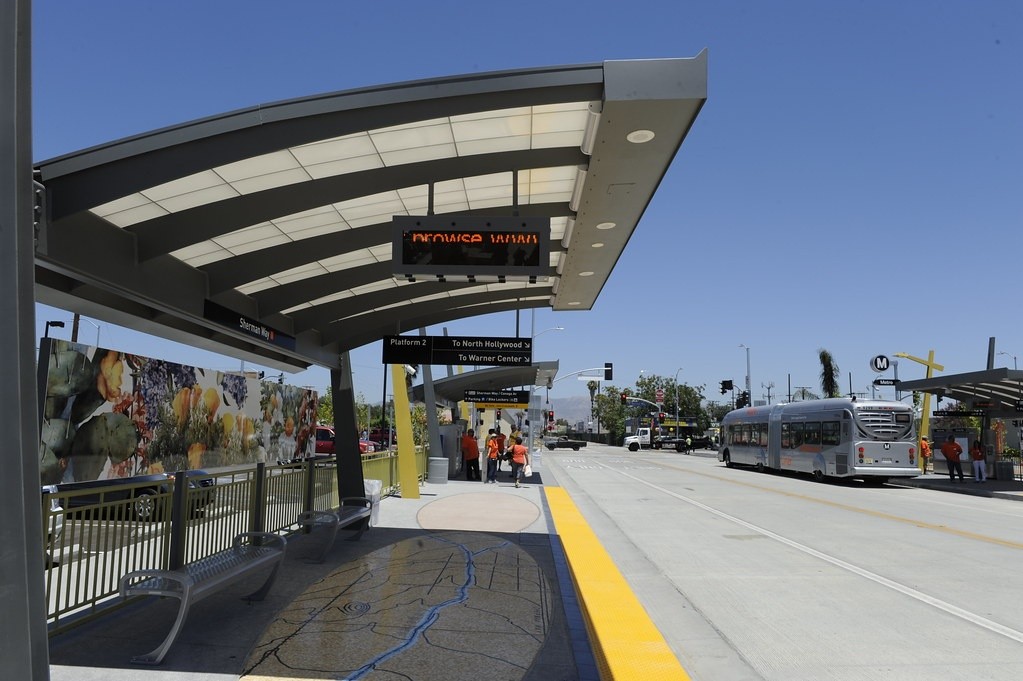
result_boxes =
[656,389,664,397]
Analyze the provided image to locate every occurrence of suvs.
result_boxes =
[277,426,396,468]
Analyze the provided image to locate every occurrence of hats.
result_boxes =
[922,435,928,438]
[491,434,499,439]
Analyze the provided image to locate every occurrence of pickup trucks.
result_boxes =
[545,436,587,451]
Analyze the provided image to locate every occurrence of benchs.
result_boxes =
[118,532,288,664]
[297,497,371,563]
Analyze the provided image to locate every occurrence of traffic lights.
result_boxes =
[741,391,749,406]
[720,380,733,395]
[548,426,551,431]
[549,411,554,421]
[621,394,626,405]
[659,413,664,424]
[497,411,501,420]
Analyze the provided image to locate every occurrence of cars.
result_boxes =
[42,470,216,549]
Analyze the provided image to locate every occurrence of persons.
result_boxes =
[941,435,966,483]
[508,423,522,477]
[363,427,367,440]
[484,429,506,483]
[967,440,987,483]
[921,435,931,474]
[507,437,529,487]
[461,429,481,481]
[685,436,691,454]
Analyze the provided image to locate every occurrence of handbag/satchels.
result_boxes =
[524,464,532,477]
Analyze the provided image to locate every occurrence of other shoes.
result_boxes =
[488,479,492,483]
[516,483,519,488]
[476,476,481,481]
[468,477,475,481]
[498,469,502,472]
[493,479,496,483]
[509,475,514,478]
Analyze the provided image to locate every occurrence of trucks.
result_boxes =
[623,412,713,451]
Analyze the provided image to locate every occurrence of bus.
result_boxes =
[715,395,922,483]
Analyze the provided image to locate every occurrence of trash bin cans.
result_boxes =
[428,457,449,484]
[995,461,1013,481]
[364,479,382,526]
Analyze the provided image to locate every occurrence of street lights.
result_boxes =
[893,349,944,474]
[738,343,751,391]
[45,321,65,338]
[996,351,1017,370]
[675,368,683,439]
